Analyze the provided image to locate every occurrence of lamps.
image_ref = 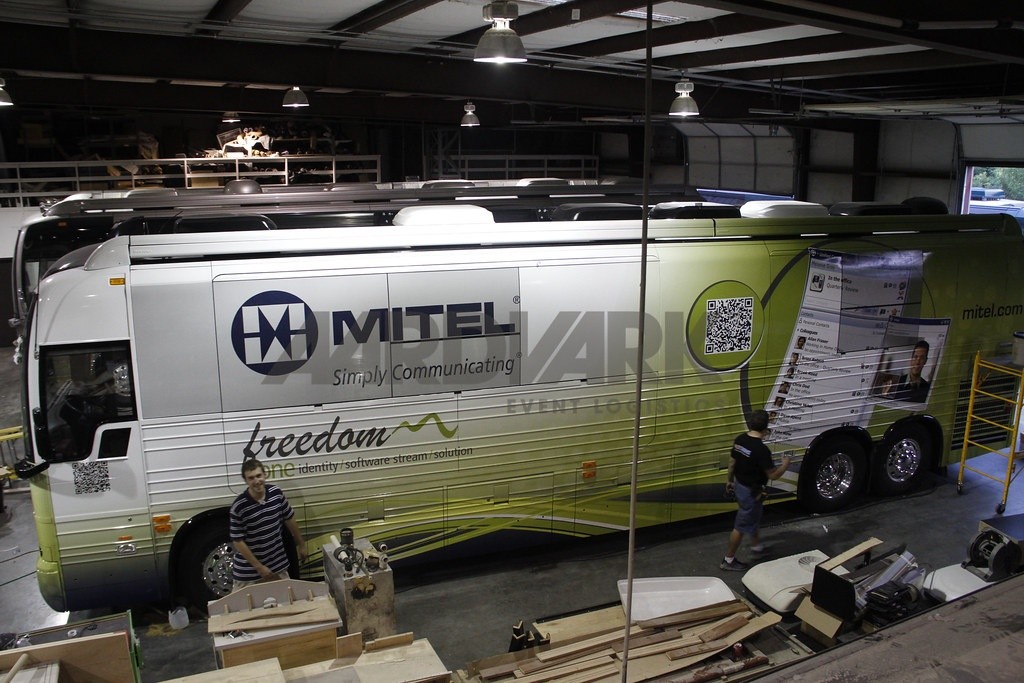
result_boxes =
[473,0,528,64]
[281,86,310,108]
[0,78,14,106]
[221,112,241,123]
[668,70,700,116]
[460,99,481,127]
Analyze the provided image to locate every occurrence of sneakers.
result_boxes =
[751,545,773,557]
[720,558,749,572]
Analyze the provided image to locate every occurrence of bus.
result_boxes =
[7,169,1024,624]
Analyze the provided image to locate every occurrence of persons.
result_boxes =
[764,430,773,440]
[811,274,823,291]
[720,410,792,573]
[230,460,310,595]
[785,368,795,379]
[769,412,778,424]
[891,341,932,404]
[796,337,807,351]
[790,354,800,366]
[779,382,791,394]
[776,397,785,407]
[874,376,897,399]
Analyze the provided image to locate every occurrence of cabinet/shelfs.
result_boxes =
[0,608,145,683]
[207,579,344,670]
[431,153,600,180]
[158,631,453,683]
